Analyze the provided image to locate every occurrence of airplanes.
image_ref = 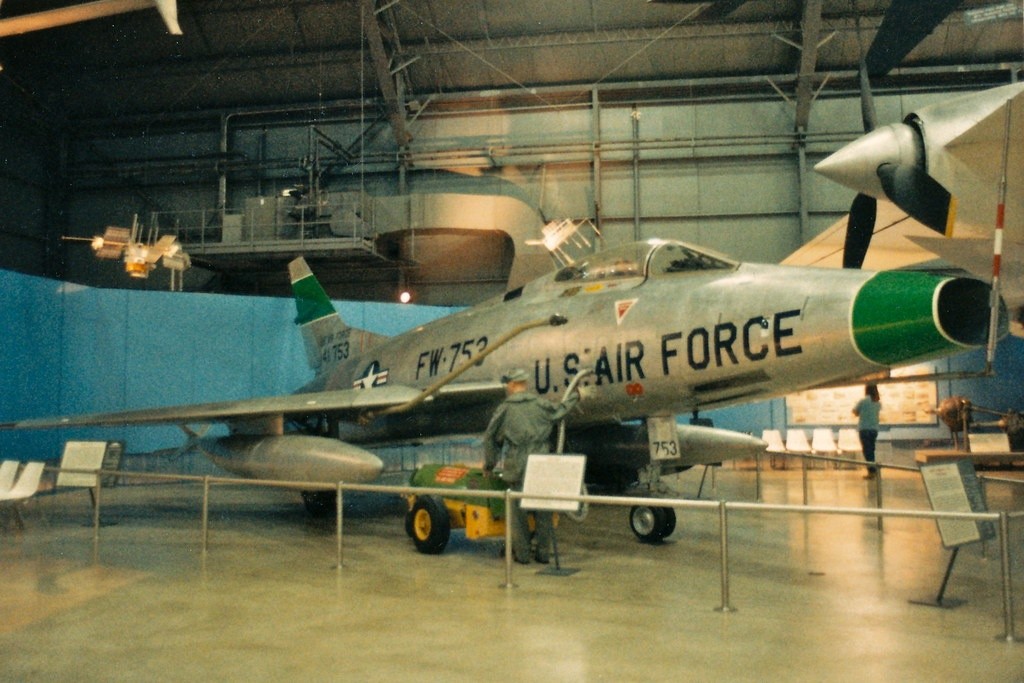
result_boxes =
[778,44,1024,340]
[1,236,1010,555]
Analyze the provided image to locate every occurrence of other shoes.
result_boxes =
[535,547,550,566]
[863,472,877,479]
[517,552,532,565]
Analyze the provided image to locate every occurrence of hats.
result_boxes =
[500,368,530,382]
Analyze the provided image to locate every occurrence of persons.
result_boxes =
[852,384,882,478]
[480,369,596,564]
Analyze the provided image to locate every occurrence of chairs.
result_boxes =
[0,460,46,531]
[761,430,863,470]
